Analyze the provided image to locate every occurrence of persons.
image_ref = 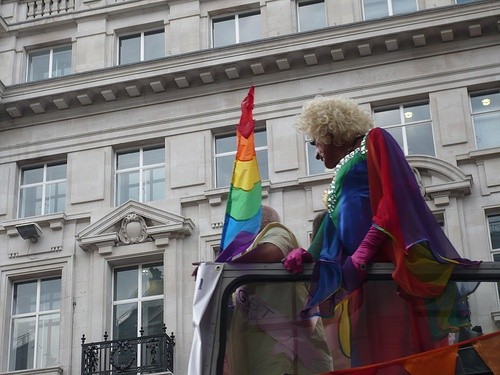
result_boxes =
[192,207,332,375]
[283,95,483,371]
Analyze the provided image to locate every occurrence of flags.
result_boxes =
[214,84,263,262]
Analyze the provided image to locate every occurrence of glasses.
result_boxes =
[310,140,316,146]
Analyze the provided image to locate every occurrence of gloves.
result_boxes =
[281,248,312,274]
[350,226,387,272]
